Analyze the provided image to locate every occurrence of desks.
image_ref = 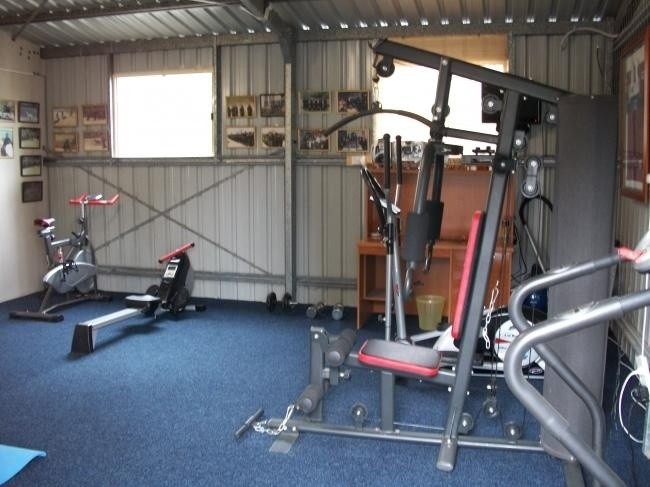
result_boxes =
[356,165,517,330]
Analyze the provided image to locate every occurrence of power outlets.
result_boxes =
[634,356,650,385]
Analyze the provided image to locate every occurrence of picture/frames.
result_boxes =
[617,21,650,208]
[0,98,110,204]
[224,89,371,154]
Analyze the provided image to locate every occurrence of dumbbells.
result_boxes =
[256,292,299,313]
[307,302,324,318]
[332,303,344,320]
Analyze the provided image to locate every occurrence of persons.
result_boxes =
[225,90,370,151]
